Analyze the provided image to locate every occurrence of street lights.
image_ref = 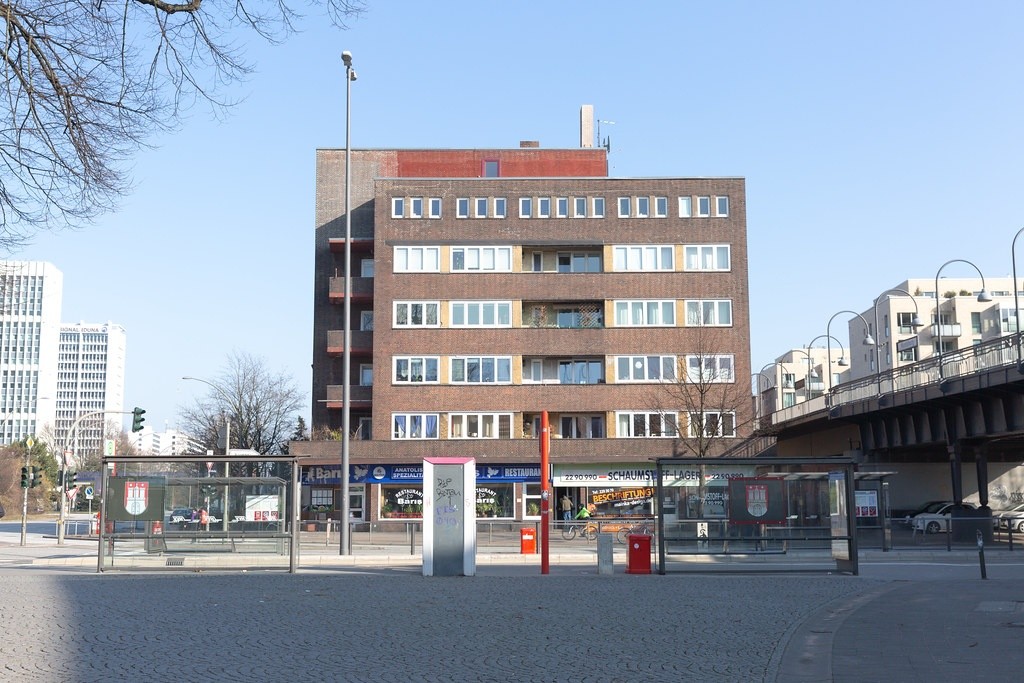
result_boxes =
[781,350,818,410]
[936,259,993,378]
[339,50,360,556]
[874,289,923,396]
[827,309,874,406]
[758,363,793,419]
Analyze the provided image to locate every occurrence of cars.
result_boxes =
[898,502,953,528]
[999,504,1024,532]
[169,507,217,524]
[992,502,1024,527]
[912,502,978,535]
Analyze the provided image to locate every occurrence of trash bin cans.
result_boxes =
[624,534,652,574]
[521,528,536,554]
[97,511,112,534]
[144,522,163,549]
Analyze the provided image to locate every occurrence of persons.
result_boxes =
[574,503,591,529]
[199,508,208,529]
[562,495,573,520]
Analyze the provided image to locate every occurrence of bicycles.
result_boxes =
[561,518,598,542]
[617,519,654,545]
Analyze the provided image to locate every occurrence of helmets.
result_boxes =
[578,504,584,508]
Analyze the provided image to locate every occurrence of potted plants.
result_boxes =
[528,503,540,516]
[476,504,503,518]
[405,506,414,518]
[415,505,423,516]
[382,504,393,518]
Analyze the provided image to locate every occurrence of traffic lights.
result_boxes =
[20,467,28,487]
[66,470,78,490]
[132,407,146,432]
[31,466,42,488]
